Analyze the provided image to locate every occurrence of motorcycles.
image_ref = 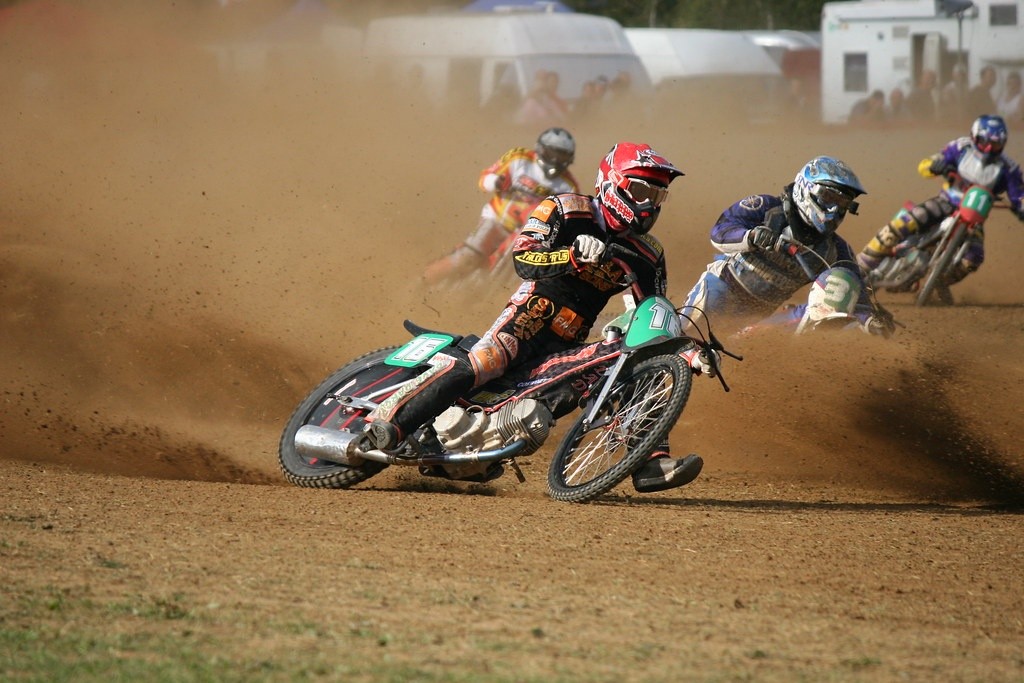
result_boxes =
[675,233,906,425]
[278,243,744,503]
[864,171,1011,307]
[428,186,547,316]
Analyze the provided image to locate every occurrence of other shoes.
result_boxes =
[937,281,953,305]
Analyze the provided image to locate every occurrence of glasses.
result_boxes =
[977,137,1003,154]
[624,178,669,207]
[547,149,569,162]
[816,186,852,215]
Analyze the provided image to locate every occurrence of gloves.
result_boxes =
[691,348,721,378]
[930,158,957,183]
[496,174,515,195]
[749,225,775,251]
[572,234,606,263]
[868,315,895,340]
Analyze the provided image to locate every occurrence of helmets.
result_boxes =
[971,115,1007,160]
[536,128,575,178]
[792,156,868,236]
[594,142,685,234]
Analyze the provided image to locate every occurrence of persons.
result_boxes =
[886,88,912,120]
[524,71,630,126]
[857,113,1024,273]
[414,127,581,293]
[942,61,967,106]
[681,156,895,352]
[907,68,938,117]
[997,73,1024,116]
[364,141,705,493]
[850,91,885,124]
[963,65,996,112]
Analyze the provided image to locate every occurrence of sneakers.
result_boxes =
[364,420,405,450]
[635,454,703,493]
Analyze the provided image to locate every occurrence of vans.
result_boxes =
[369,0,821,118]
